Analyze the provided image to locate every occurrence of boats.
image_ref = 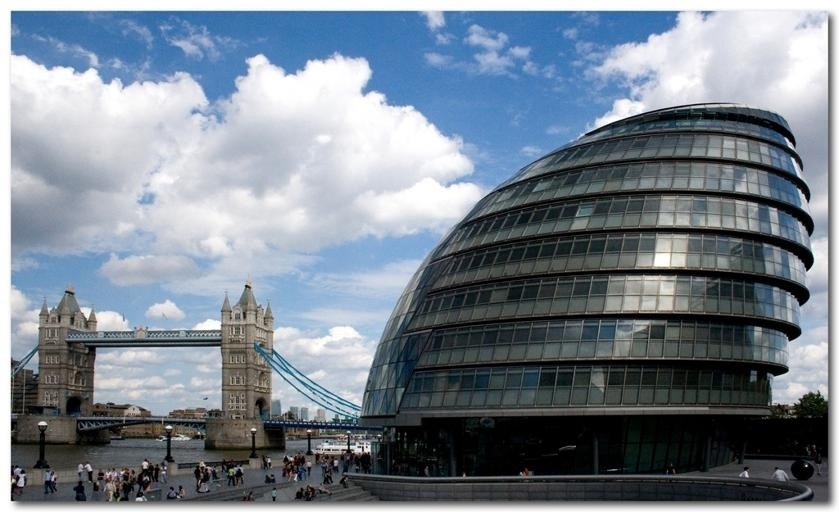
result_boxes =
[156,434,192,441]
[314,440,370,455]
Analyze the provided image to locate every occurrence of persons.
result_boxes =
[11,449,372,501]
[803,442,822,457]
[738,466,749,486]
[770,466,789,481]
[814,451,822,475]
[666,463,676,474]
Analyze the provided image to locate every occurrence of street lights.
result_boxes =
[376,434,382,442]
[248,427,259,459]
[34,420,51,469]
[163,424,175,462]
[306,430,313,455]
[346,430,353,453]
